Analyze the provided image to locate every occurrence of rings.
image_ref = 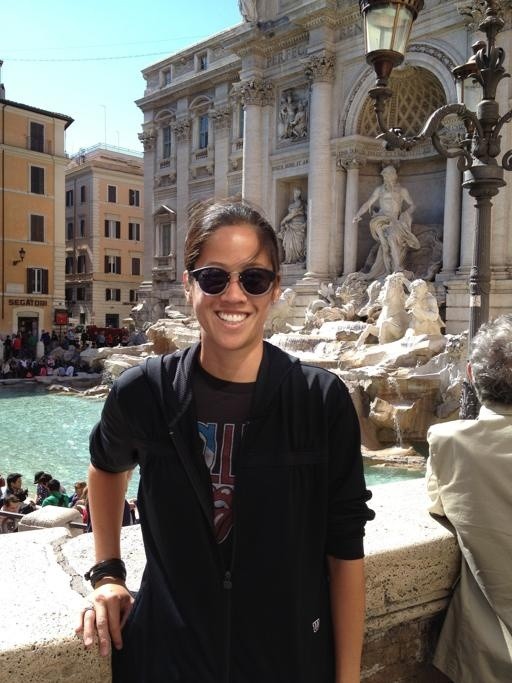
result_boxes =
[84,602,96,613]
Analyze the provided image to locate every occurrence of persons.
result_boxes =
[276,188,307,264]
[278,93,308,139]
[351,165,420,274]
[75,194,374,683]
[0,471,92,533]
[425,315,512,682]
[0,324,148,379]
[355,271,446,349]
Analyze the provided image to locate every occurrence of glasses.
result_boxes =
[188,268,277,296]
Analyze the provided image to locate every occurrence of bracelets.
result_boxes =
[84,558,126,588]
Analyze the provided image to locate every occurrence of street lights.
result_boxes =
[362,1,512,424]
